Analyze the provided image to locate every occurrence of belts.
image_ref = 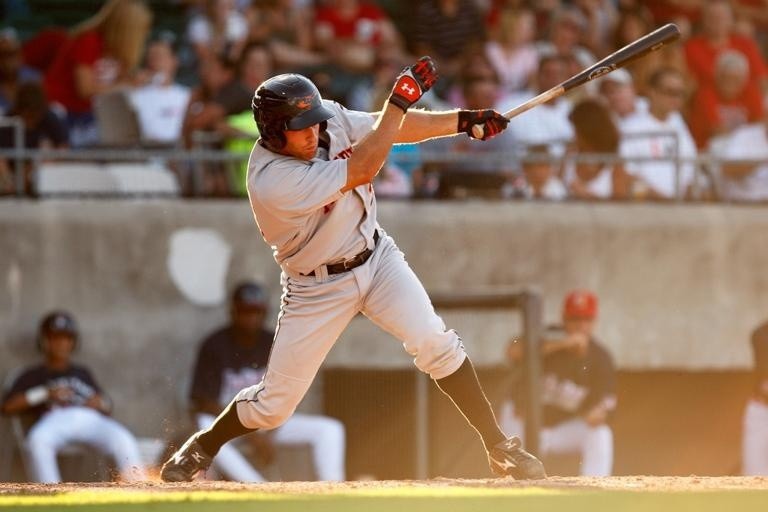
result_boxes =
[300,230,380,277]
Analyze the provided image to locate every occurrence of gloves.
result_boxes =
[387,56,440,114]
[456,107,511,141]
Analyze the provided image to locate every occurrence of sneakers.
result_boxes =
[160,429,214,483]
[487,436,546,480]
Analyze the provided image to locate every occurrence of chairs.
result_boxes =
[3,364,112,483]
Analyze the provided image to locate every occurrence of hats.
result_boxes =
[234,283,268,313]
[562,288,598,321]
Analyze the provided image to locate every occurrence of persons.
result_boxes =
[741,319,766,473]
[497,292,618,475]
[0,309,147,484]
[1,1,768,204]
[161,56,552,484]
[187,282,349,484]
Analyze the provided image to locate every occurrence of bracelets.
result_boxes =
[24,381,51,406]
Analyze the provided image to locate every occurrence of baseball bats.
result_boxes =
[471,23,679,138]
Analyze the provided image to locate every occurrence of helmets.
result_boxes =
[39,313,77,340]
[251,72,337,154]
